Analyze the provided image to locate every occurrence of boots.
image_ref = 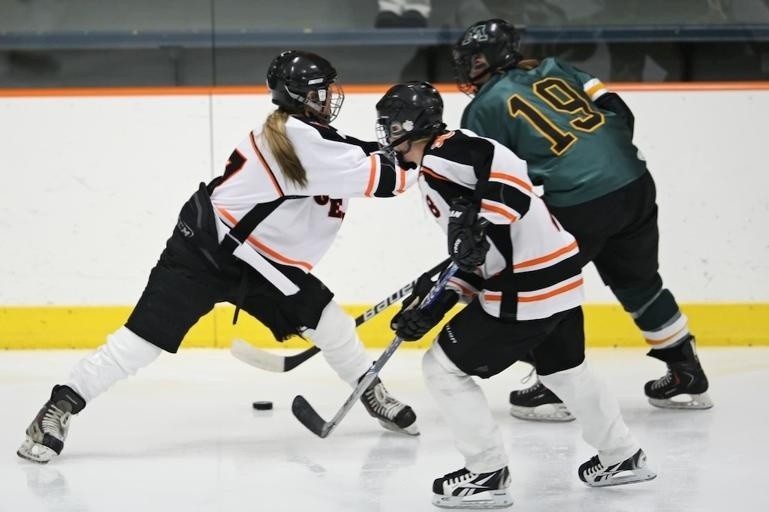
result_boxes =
[644,337,707,399]
[26,383,86,455]
[360,361,416,429]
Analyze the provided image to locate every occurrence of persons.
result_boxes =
[451,16,709,408]
[374,80,650,498]
[15,48,417,462]
[374,1,433,30]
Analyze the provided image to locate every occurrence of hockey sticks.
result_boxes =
[230,255,459,439]
[292,216,495,440]
[229,256,458,374]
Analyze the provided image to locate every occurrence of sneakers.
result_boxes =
[509,383,563,407]
[578,449,648,483]
[432,468,512,496]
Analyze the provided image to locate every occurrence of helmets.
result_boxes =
[451,18,520,94]
[267,49,345,123]
[374,80,444,168]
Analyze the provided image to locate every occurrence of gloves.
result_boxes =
[390,272,461,342]
[446,201,490,274]
